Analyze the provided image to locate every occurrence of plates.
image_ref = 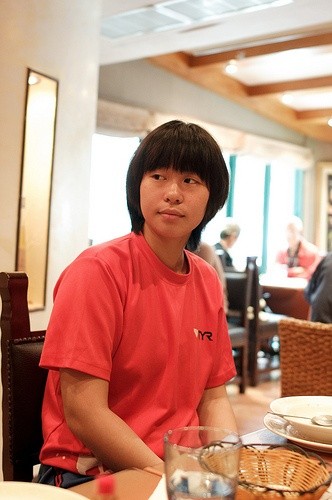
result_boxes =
[263,413,332,453]
[0,481,89,500]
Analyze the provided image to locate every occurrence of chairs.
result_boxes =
[220,256,332,399]
[3,339,52,479]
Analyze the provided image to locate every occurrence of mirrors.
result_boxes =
[14,67,59,314]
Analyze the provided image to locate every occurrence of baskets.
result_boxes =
[199,440,332,500]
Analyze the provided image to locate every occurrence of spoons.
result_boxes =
[267,411,332,427]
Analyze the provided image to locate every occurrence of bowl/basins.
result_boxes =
[270,396,332,443]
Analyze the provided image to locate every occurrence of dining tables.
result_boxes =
[67,428,332,500]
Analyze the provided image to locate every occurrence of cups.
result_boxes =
[163,425,243,500]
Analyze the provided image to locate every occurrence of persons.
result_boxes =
[211,216,282,356]
[40,121,244,491]
[303,251,332,324]
[191,239,230,317]
[274,216,321,281]
